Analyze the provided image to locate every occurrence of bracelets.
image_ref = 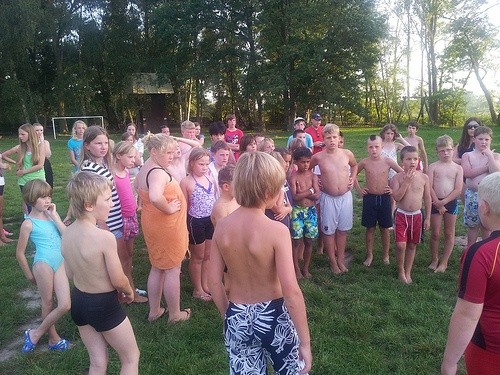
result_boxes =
[309,188,314,195]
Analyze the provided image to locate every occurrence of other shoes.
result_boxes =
[3,229,14,236]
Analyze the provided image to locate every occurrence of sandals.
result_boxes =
[148,306,168,324]
[48,338,76,351]
[22,328,36,352]
[168,308,190,322]
[193,292,213,301]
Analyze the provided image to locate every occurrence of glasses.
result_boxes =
[467,124,479,129]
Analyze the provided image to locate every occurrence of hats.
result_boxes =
[311,114,323,121]
[294,117,306,124]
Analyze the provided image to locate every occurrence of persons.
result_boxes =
[2,123,54,218]
[405,122,429,171]
[0,153,14,243]
[354,135,404,267]
[15,178,73,354]
[63,115,351,302]
[379,123,412,216]
[59,171,141,375]
[440,171,500,375]
[392,146,432,285]
[461,126,500,248]
[134,130,192,322]
[308,123,359,275]
[452,117,485,204]
[207,150,312,375]
[425,133,464,273]
